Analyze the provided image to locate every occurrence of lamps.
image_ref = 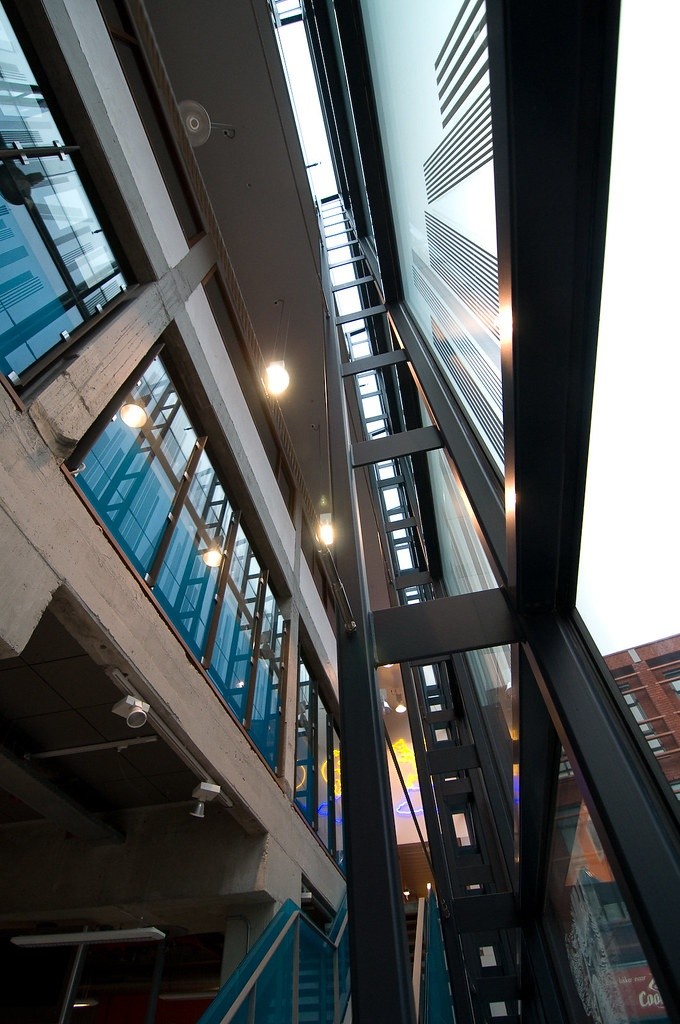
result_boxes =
[190,782,221,817]
[180,100,236,148]
[202,534,224,568]
[395,694,406,714]
[264,297,293,396]
[110,695,150,728]
[382,700,392,715]
[0,164,77,206]
[119,399,148,429]
